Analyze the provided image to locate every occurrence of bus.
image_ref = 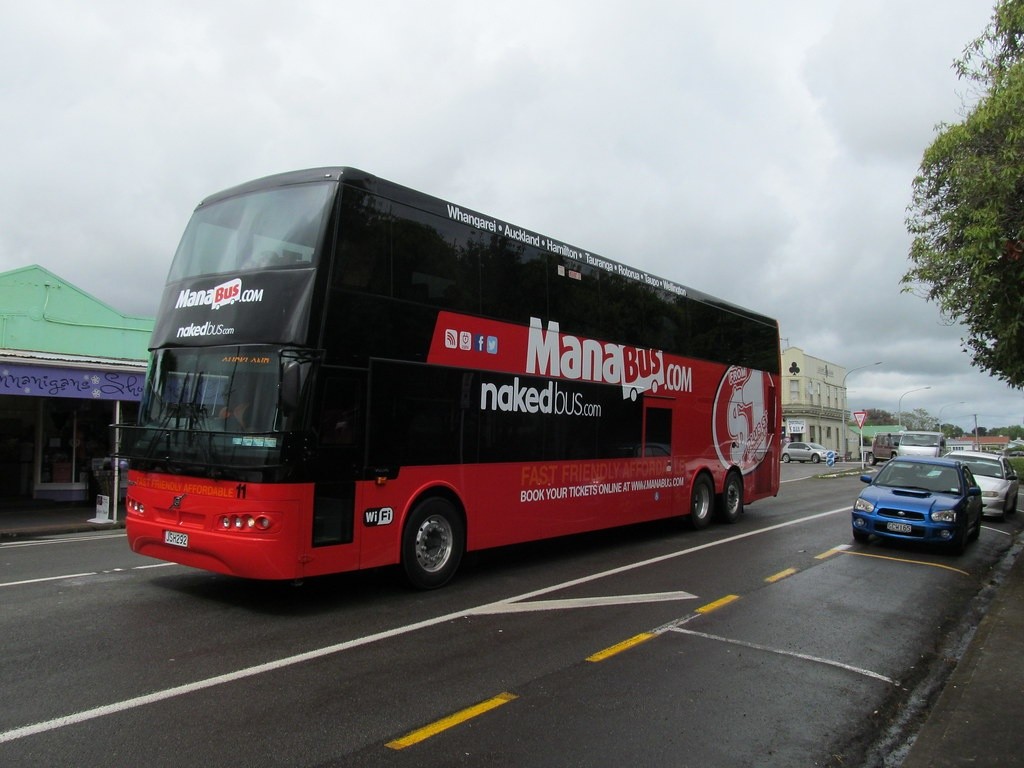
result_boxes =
[107,165,783,593]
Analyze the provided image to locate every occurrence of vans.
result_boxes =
[894,430,946,458]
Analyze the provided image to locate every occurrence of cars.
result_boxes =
[927,450,1019,523]
[780,442,840,464]
[852,454,983,556]
[1009,450,1024,456]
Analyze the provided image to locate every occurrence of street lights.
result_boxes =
[939,401,965,432]
[842,361,883,464]
[899,387,932,431]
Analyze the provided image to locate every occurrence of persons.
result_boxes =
[219,384,252,428]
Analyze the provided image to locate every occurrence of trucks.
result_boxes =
[857,432,901,466]
